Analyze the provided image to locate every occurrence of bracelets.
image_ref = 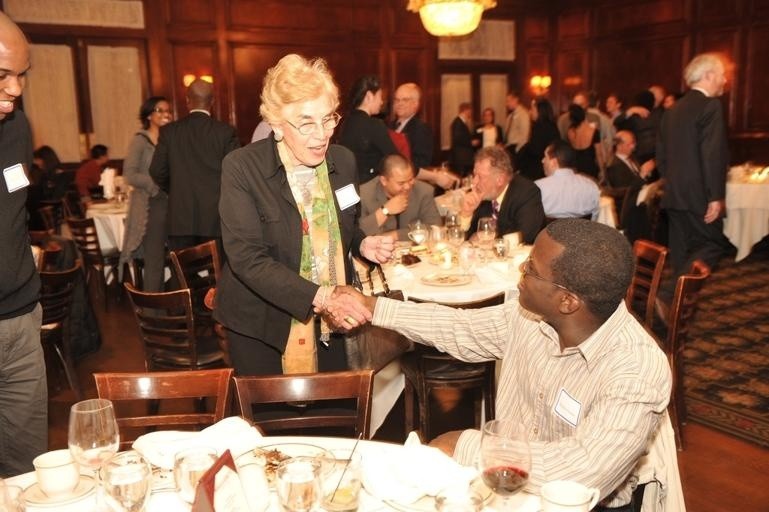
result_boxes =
[322,284,329,310]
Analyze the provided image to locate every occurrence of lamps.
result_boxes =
[406,0,497,39]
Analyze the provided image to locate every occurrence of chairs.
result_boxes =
[91,367,232,452]
[649,261,712,449]
[399,290,508,445]
[232,368,374,439]
[623,237,670,347]
[66,217,144,311]
[168,239,224,360]
[121,279,230,435]
[36,259,85,404]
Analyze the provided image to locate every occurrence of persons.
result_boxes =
[313,219,686,512]
[558,92,615,166]
[606,93,623,120]
[503,90,531,155]
[448,103,478,179]
[149,79,245,342]
[517,97,560,180]
[566,103,605,185]
[663,94,677,110]
[655,53,729,300]
[614,86,664,130]
[607,131,657,226]
[76,144,108,198]
[453,147,547,246]
[630,90,656,156]
[33,142,70,185]
[531,140,601,223]
[120,96,173,316]
[389,82,436,183]
[358,154,442,243]
[474,108,503,149]
[339,77,461,190]
[210,53,395,438]
[0,14,48,478]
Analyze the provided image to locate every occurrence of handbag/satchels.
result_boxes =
[343,244,412,375]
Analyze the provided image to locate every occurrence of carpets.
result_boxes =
[632,241,768,452]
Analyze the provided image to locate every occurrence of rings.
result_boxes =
[343,316,354,326]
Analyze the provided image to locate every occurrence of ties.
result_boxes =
[625,158,639,176]
[505,113,514,142]
[487,201,501,229]
[394,121,401,132]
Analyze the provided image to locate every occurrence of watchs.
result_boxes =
[381,207,390,217]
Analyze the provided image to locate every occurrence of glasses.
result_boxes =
[524,258,572,296]
[154,107,172,114]
[283,111,342,135]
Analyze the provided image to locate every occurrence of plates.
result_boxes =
[359,483,496,512]
[16,474,98,508]
[232,441,339,482]
[419,270,473,287]
[131,430,216,473]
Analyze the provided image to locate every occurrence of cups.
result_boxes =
[320,448,365,512]
[100,449,153,512]
[114,193,126,210]
[456,246,476,276]
[447,227,466,262]
[477,419,533,510]
[428,229,447,265]
[31,448,82,500]
[477,217,498,266]
[410,223,427,258]
[273,455,327,511]
[444,209,463,227]
[492,237,510,281]
[537,480,601,512]
[406,229,427,244]
[67,398,121,512]
